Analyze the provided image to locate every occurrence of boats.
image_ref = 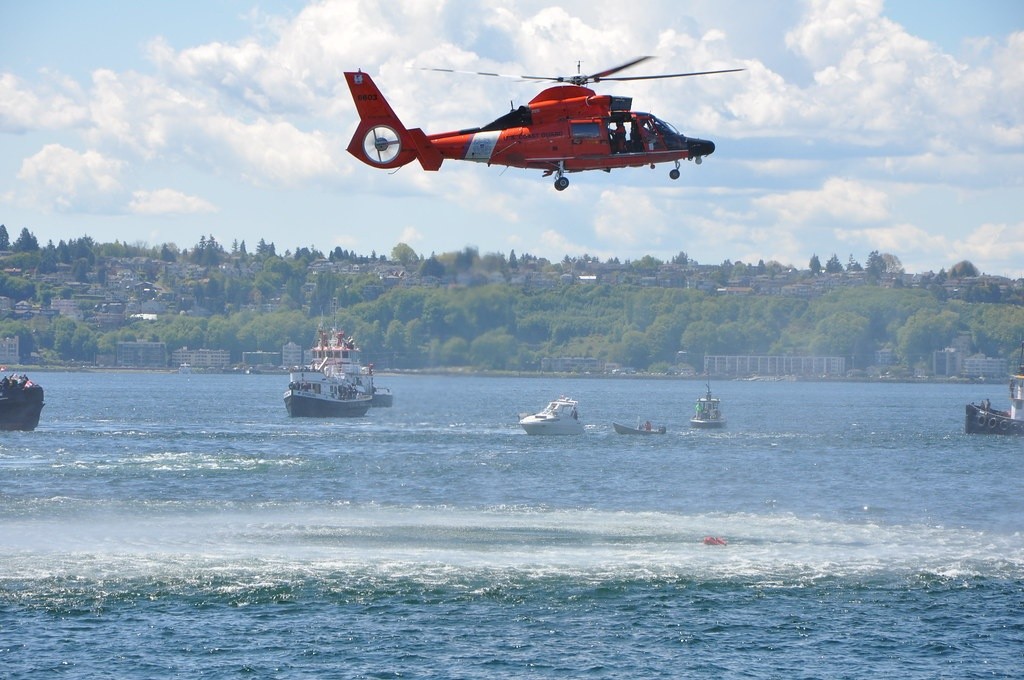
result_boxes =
[515,395,587,435]
[690,371,729,430]
[612,415,666,436]
[965,339,1024,435]
[282,295,373,419]
[0,380,46,434]
[370,387,395,408]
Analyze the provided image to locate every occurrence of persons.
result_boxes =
[1,375,9,384]
[644,420,651,431]
[695,403,702,420]
[571,407,577,420]
[22,373,29,381]
[608,120,626,154]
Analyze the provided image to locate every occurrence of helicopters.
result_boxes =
[342,53,746,190]
